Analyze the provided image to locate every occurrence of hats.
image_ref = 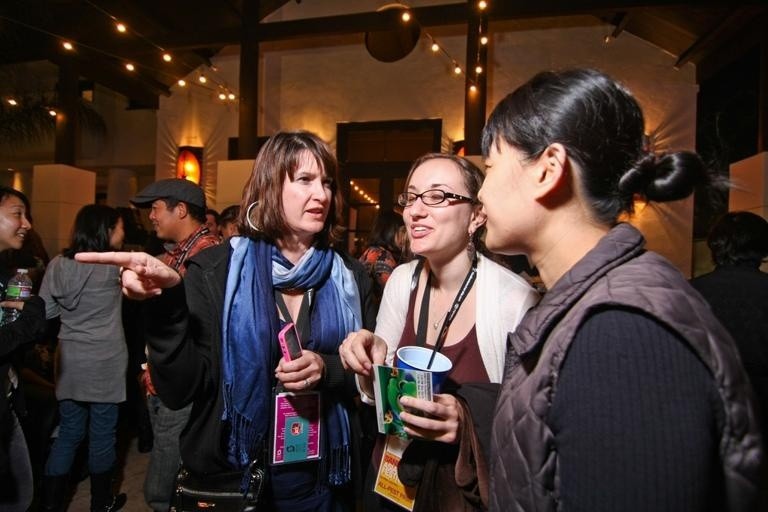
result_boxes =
[128,177,207,210]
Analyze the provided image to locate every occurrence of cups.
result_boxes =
[395,345,453,394]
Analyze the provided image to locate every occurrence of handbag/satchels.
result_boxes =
[168,456,267,512]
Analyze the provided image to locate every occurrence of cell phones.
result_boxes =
[278,322,303,364]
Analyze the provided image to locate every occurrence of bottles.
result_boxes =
[3,267,32,321]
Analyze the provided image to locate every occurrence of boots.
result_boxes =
[89,469,127,512]
[38,473,70,512]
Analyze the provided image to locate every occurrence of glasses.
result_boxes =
[397,188,478,207]
